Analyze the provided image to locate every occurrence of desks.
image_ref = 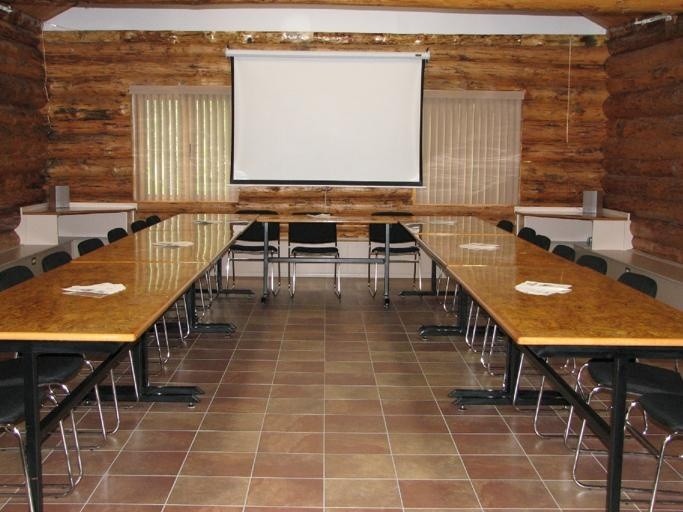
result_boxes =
[397,216,682,509]
[0,200,139,292]
[256,215,398,309]
[1,211,257,512]
[514,204,683,315]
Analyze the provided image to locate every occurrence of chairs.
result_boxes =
[621,394,683,512]
[287,212,341,298]
[452,227,537,325]
[77,238,184,364]
[564,358,683,497]
[107,227,194,339]
[0,266,140,436]
[465,244,576,353]
[367,211,423,298]
[42,251,164,387]
[462,235,551,346]
[225,209,281,297]
[2,354,108,485]
[130,219,206,321]
[481,255,607,377]
[0,388,75,512]
[146,214,221,308]
[511,273,658,439]
[436,219,515,314]
